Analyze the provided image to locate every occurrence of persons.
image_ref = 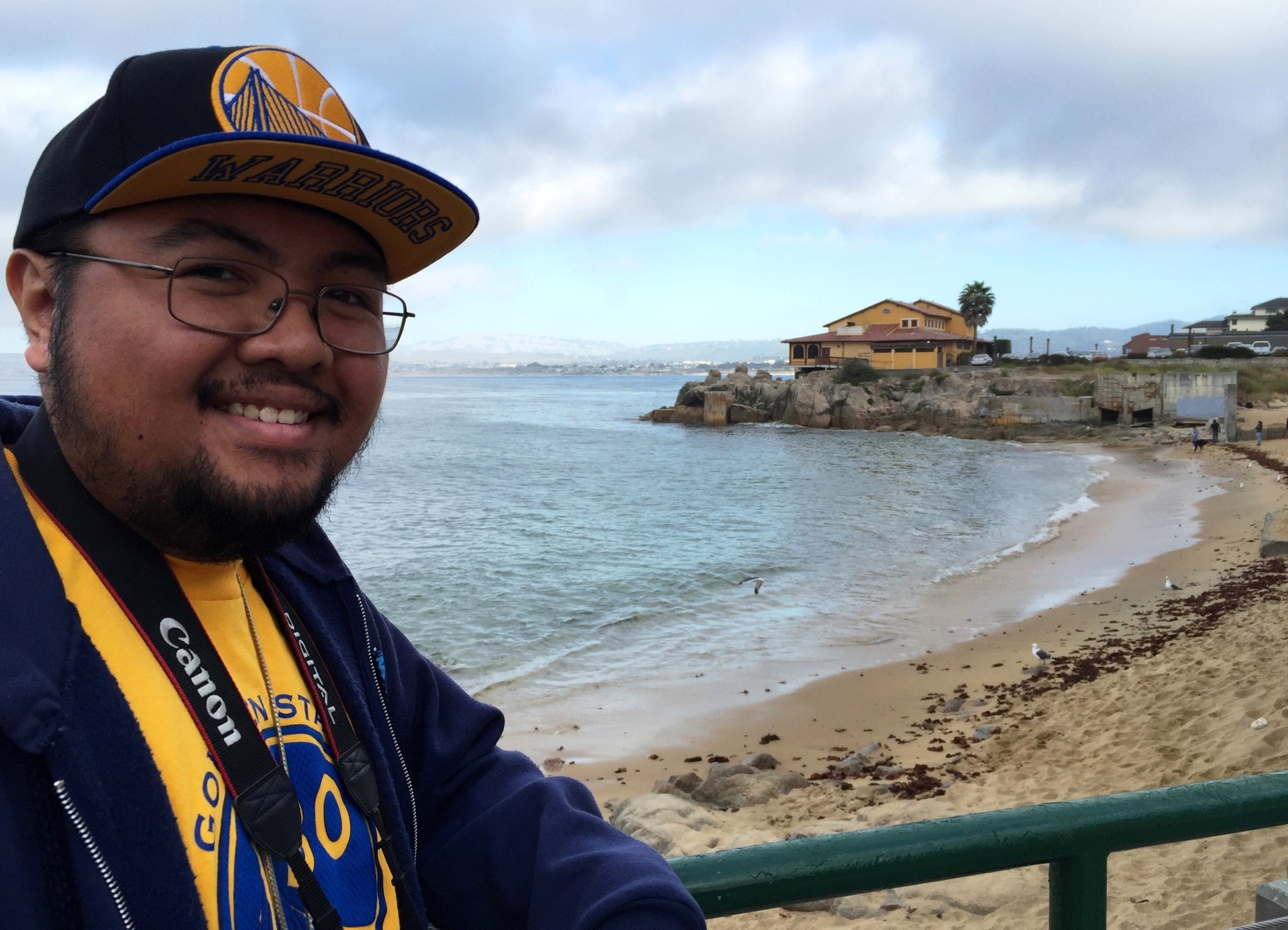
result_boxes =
[1253,421,1263,446]
[0,44,703,930]
[1191,425,1200,450]
[1209,419,1220,445]
[1192,439,1208,452]
[1285,418,1288,439]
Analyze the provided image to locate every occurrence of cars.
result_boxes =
[1172,343,1213,358]
[1146,346,1160,359]
[1153,347,1172,359]
[971,353,993,364]
[1224,340,1288,356]
[1026,348,1111,364]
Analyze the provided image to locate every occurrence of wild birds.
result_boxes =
[1165,576,1183,594]
[736,576,765,595]
[1031,643,1056,665]
[1239,464,1282,489]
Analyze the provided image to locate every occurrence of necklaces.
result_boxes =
[238,571,315,930]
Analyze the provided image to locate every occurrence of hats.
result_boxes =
[10,46,481,284]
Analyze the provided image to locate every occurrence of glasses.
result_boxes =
[38,248,415,357]
